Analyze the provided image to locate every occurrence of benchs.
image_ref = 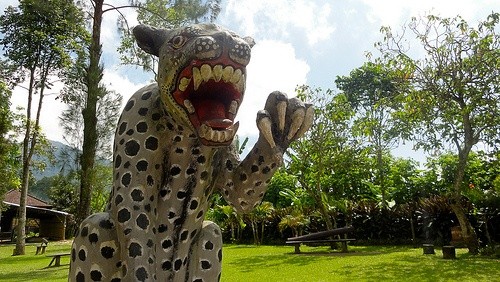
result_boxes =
[286,225,356,253]
[12,225,40,243]
[35,238,48,254]
[45,252,71,267]
